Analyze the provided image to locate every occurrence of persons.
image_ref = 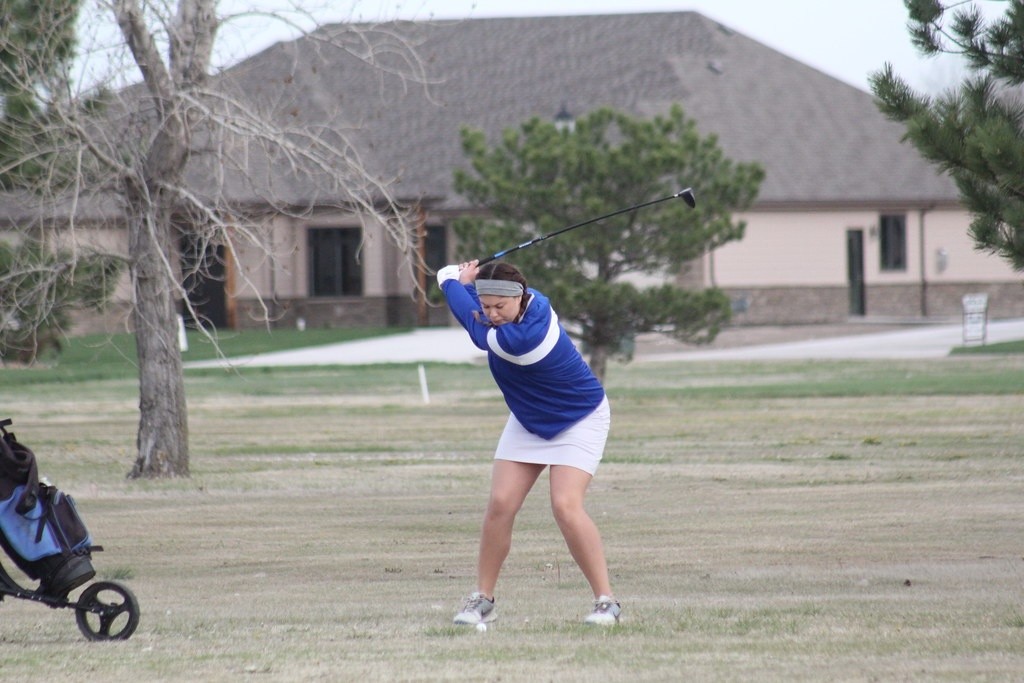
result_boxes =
[438,259,619,625]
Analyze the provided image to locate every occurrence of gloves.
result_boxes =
[436,265,467,290]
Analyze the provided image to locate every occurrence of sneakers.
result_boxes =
[452,595,498,624]
[582,596,623,625]
